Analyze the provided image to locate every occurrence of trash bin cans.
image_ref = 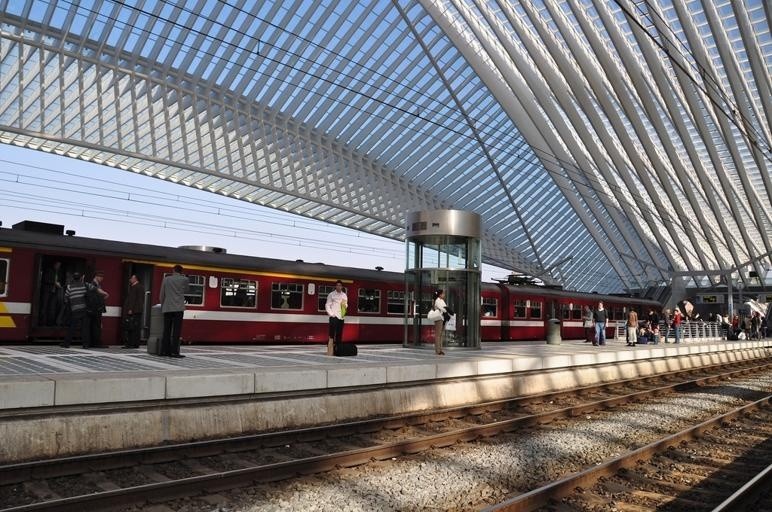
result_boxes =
[147,304,173,355]
[547,318,561,344]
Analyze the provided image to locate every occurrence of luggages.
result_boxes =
[590,320,602,345]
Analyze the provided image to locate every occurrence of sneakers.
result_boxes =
[60,343,69,348]
[83,342,89,348]
[159,351,185,357]
[122,344,139,349]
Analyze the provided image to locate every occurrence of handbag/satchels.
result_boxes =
[335,343,357,356]
[427,309,442,321]
[123,313,141,328]
[84,287,105,312]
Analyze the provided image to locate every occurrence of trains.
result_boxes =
[0,219,661,347]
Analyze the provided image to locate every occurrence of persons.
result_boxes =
[592,300,609,347]
[158,264,190,358]
[621,304,768,348]
[120,271,145,350]
[432,288,454,356]
[39,255,111,351]
[582,303,594,344]
[324,279,350,357]
